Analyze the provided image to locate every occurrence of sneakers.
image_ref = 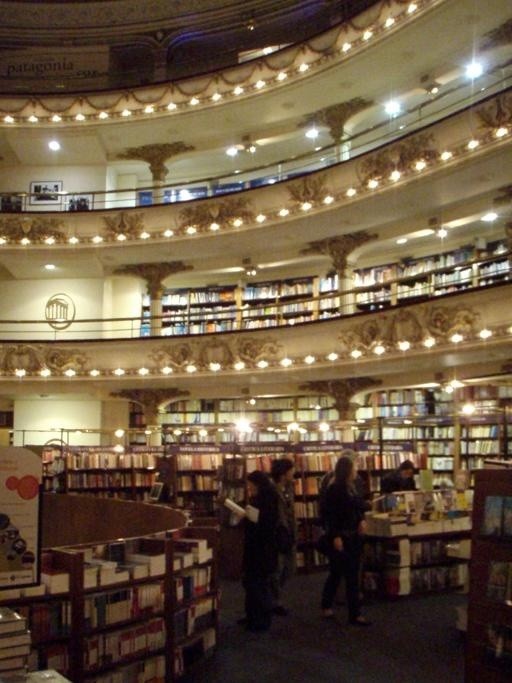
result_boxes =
[273,605,289,616]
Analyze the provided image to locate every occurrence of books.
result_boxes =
[139,239,512,337]
[482,562,512,660]
[42,380,512,567]
[1,538,223,683]
[359,564,468,596]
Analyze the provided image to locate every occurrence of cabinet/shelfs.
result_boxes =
[0,385,512,683]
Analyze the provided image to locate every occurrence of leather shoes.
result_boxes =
[349,619,377,626]
[325,615,337,620]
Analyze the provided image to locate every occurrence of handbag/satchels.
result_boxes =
[272,525,289,553]
[316,535,352,560]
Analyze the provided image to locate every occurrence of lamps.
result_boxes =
[2,3,422,131]
[2,121,512,251]
[3,323,512,379]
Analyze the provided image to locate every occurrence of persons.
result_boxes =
[235,457,297,640]
[77,200,88,211]
[318,453,375,625]
